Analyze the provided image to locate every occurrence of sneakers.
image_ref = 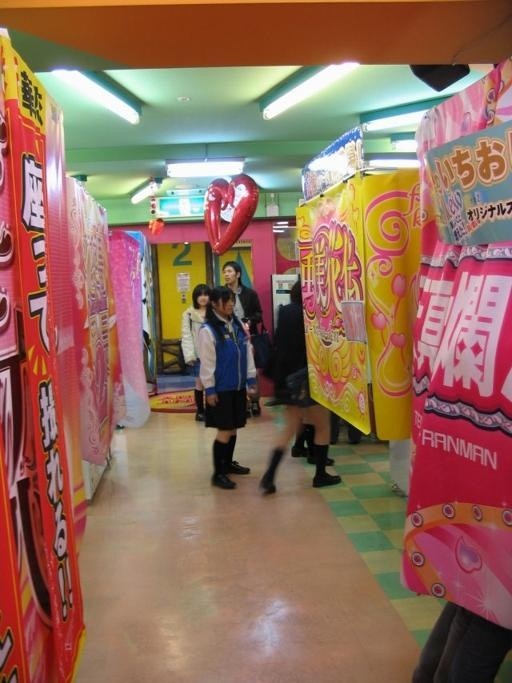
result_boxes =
[260,446,341,493]
[245,398,261,418]
[195,406,207,423]
[211,461,250,490]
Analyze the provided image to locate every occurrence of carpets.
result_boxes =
[143,386,253,414]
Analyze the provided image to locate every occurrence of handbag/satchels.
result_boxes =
[251,331,274,368]
[269,351,301,389]
[286,365,320,407]
[177,342,188,369]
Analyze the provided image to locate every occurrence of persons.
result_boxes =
[291,407,337,466]
[401,597,511,681]
[181,283,212,420]
[221,260,266,420]
[195,283,257,488]
[259,276,344,494]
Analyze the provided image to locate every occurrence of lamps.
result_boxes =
[160,157,248,178]
[254,60,361,124]
[48,64,143,127]
[357,88,469,171]
[128,177,164,207]
